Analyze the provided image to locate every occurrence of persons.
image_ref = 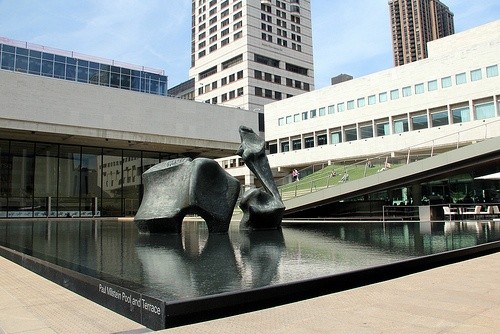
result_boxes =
[341,172,349,182]
[293,170,297,183]
[393,192,500,207]
[368,160,375,168]
[330,172,336,177]
[378,162,392,172]
[294,169,299,182]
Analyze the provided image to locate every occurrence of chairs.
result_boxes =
[443,204,500,222]
